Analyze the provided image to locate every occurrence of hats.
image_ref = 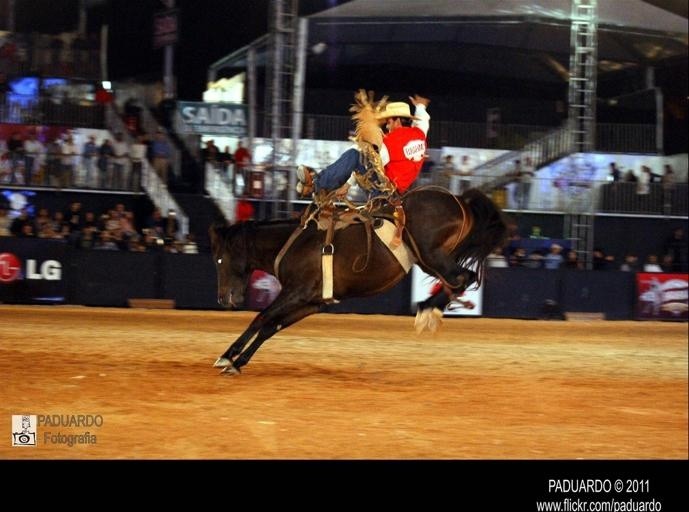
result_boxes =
[374,102,422,121]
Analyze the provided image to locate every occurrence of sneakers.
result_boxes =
[296,164,313,195]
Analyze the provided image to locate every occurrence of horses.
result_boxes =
[209,187,508,376]
